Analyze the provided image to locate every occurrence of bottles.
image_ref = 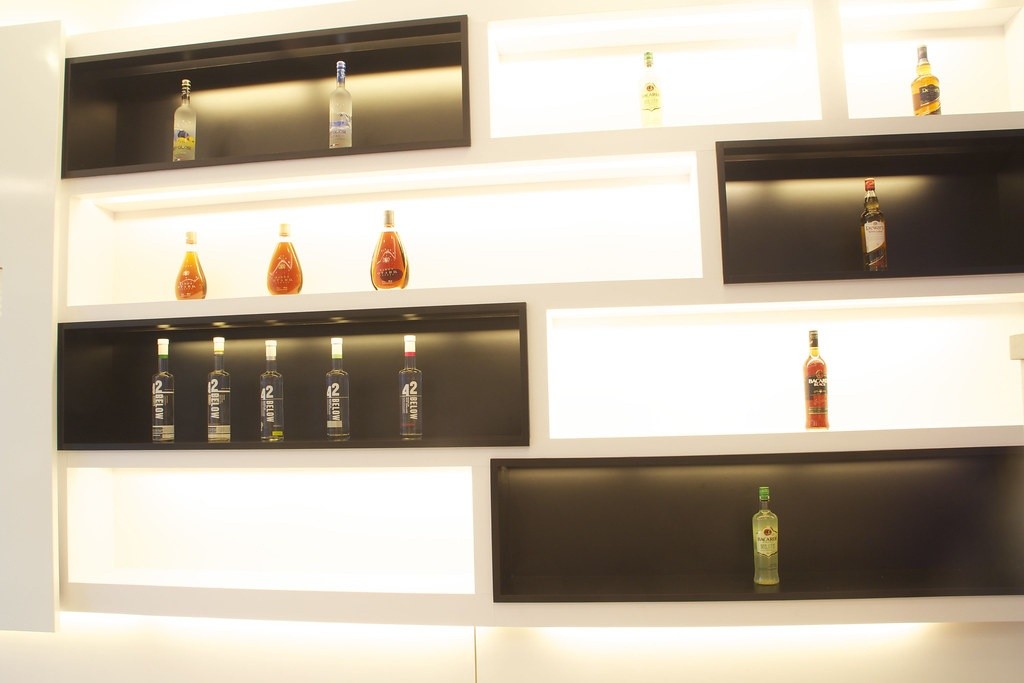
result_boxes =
[207,337,231,443]
[175,231,207,300]
[260,340,284,441]
[640,52,660,128]
[326,338,350,439]
[329,61,352,148]
[173,79,196,161]
[860,178,888,272]
[267,222,302,295]
[370,210,409,290]
[151,338,175,442]
[803,331,829,430]
[751,487,779,585]
[399,335,423,438]
[911,45,941,115]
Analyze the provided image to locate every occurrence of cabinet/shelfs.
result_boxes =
[55,0,1024,628]
